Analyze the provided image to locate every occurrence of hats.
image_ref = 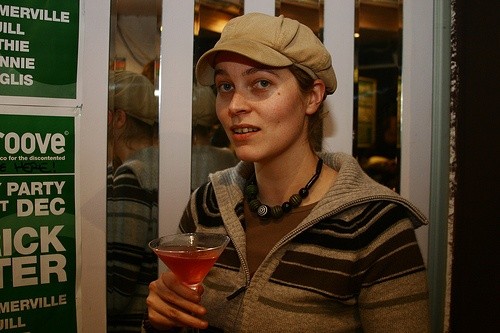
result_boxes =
[192,84,218,127]
[196,11,337,95]
[108,71,158,123]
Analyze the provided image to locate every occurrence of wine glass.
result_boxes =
[149,234,230,333]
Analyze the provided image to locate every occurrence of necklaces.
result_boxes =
[243,157,324,221]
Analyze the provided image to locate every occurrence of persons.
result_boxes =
[106,68,236,333]
[142,13,430,333]
